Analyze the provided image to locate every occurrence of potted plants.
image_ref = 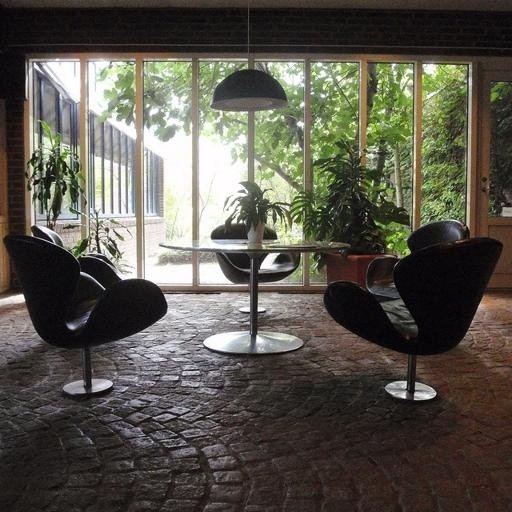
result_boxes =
[290,136,410,296]
[225,182,292,238]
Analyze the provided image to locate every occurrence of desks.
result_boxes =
[160,240,351,357]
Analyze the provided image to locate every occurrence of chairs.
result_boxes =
[368,221,472,300]
[3,232,170,397]
[210,222,302,313]
[33,225,123,277]
[321,237,504,400]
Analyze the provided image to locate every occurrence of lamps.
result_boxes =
[210,1,288,111]
